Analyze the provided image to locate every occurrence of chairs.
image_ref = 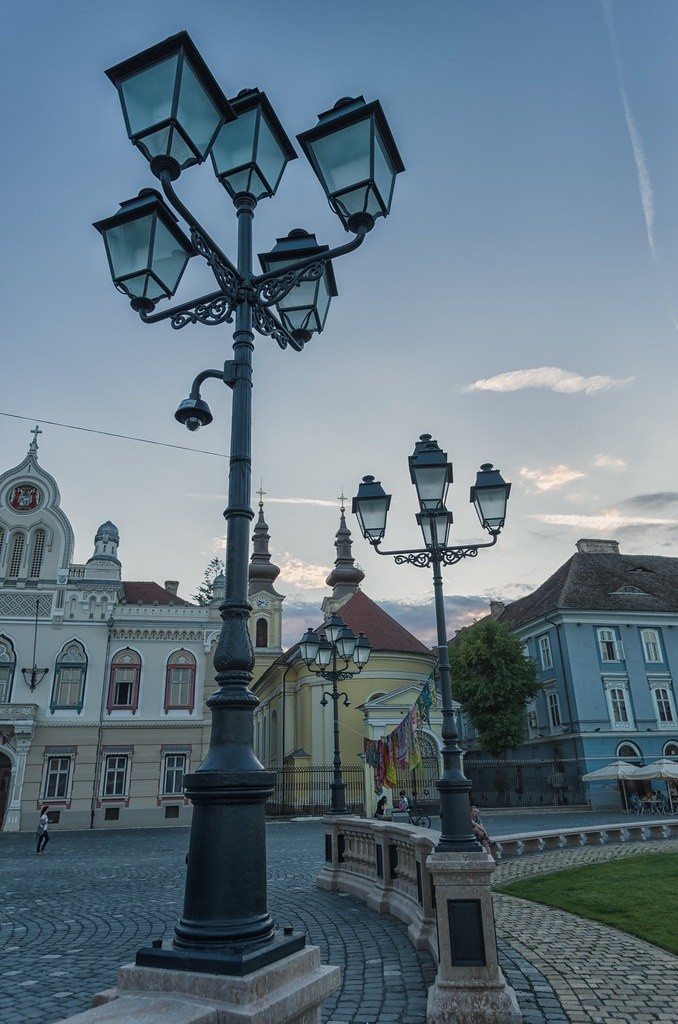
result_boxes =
[633,799,667,816]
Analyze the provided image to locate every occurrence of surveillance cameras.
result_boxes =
[343,701,351,707]
[319,700,328,706]
[174,399,214,432]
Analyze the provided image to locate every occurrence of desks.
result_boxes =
[640,801,661,816]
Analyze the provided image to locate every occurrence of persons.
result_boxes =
[630,791,668,814]
[37,806,53,855]
[471,806,494,856]
[399,790,410,813]
[375,796,388,819]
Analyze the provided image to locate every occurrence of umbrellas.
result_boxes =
[582,761,640,813]
[629,759,678,813]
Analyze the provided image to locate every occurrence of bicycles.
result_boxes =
[406,807,432,829]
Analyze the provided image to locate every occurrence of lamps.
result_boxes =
[538,733,545,737]
[647,728,654,732]
[105,614,114,642]
[595,728,601,733]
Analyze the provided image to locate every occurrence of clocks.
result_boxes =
[256,597,269,608]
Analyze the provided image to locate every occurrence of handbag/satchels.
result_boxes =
[37,825,45,835]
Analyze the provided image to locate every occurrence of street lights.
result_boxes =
[299,610,373,815]
[85,22,412,978]
[348,433,514,853]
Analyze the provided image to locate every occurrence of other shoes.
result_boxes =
[487,839,496,846]
[38,850,46,855]
